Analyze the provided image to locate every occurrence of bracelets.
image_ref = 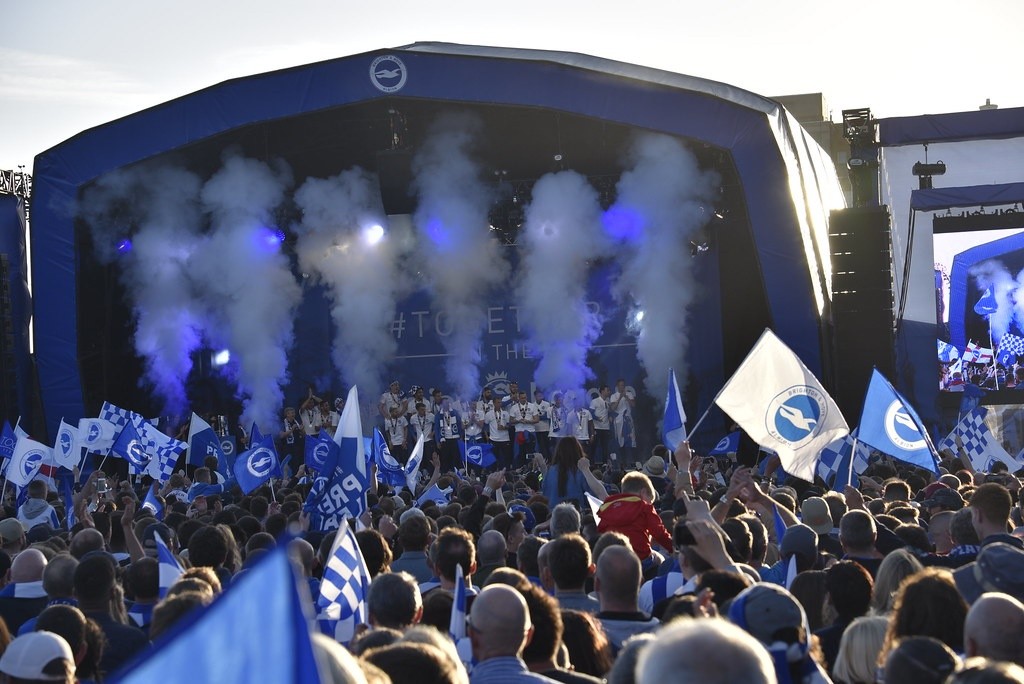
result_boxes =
[957,447,963,452]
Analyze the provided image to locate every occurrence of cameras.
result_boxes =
[526,454,535,459]
[98,479,112,494]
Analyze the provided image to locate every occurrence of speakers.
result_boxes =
[829,204,898,434]
[0,254,20,437]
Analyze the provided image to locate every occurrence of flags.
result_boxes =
[449,566,465,644]
[709,432,739,455]
[300,385,424,531]
[940,408,1022,474]
[0,402,288,529]
[759,447,777,456]
[857,368,942,476]
[110,536,333,684]
[663,369,686,451]
[937,284,1024,376]
[815,427,874,493]
[155,533,186,602]
[715,328,850,482]
[458,439,496,468]
[585,493,604,526]
[315,520,373,648]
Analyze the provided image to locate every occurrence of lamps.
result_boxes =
[846,158,869,174]
[912,162,947,177]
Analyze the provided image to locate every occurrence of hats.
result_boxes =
[670,520,695,548]
[780,524,818,565]
[0,630,74,681]
[392,495,406,510]
[801,497,835,534]
[974,542,1024,603]
[0,518,23,542]
[727,582,812,647]
[509,505,537,530]
[400,507,425,524]
[641,456,669,480]
[249,496,269,515]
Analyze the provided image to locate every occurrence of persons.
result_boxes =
[0,438,1024,684]
[939,346,1024,390]
[238,381,637,474]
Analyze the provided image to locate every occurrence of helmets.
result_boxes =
[923,482,950,500]
